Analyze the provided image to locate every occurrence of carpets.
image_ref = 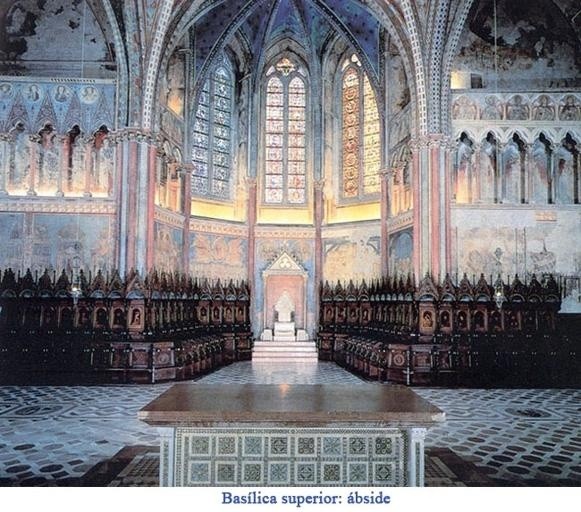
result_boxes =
[84,446,493,487]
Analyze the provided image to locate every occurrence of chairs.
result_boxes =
[273,290,295,337]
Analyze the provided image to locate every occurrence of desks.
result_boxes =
[140,383,446,487]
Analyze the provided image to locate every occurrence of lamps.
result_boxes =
[68,0,86,306]
[492,0,508,309]
